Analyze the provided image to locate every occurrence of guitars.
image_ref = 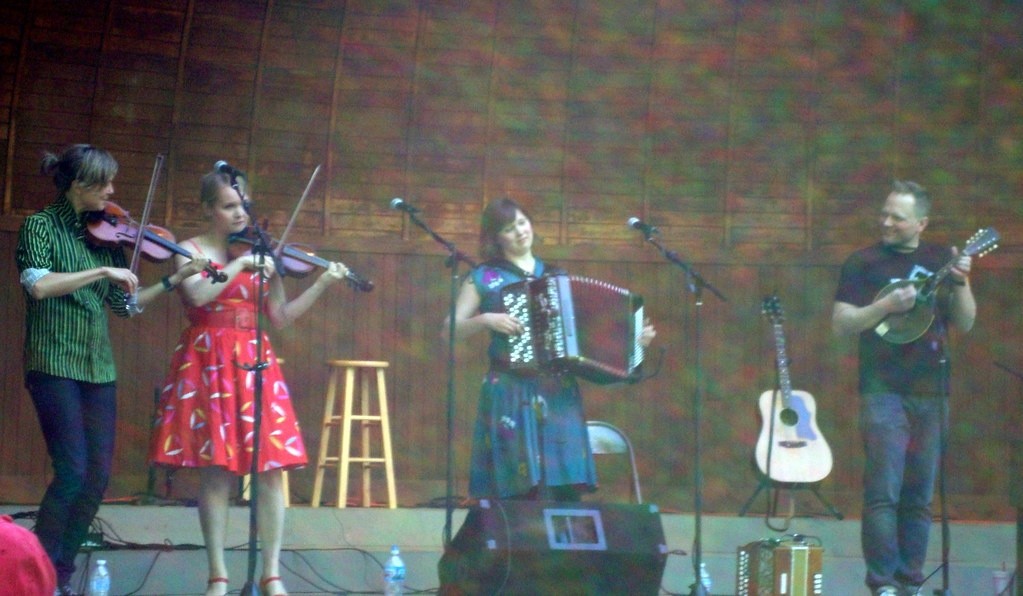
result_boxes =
[870,224,1001,345]
[752,294,835,486]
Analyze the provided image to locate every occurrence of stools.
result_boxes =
[310,359,398,510]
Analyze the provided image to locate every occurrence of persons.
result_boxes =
[832,180,976,596]
[0,144,210,595]
[436,198,656,504]
[149,169,347,596]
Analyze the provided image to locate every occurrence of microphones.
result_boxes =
[629,217,661,237]
[390,198,419,214]
[213,161,243,176]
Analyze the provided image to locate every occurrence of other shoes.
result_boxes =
[872,585,901,596]
[54,581,78,596]
[205,578,228,596]
[259,575,289,596]
[902,585,923,596]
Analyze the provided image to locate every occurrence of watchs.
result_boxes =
[162,275,177,291]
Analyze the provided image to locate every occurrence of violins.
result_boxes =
[84,199,230,285]
[228,225,377,294]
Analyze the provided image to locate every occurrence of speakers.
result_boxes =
[437,496,665,596]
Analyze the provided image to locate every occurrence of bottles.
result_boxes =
[89,560,111,596]
[383,545,407,596]
[694,563,712,596]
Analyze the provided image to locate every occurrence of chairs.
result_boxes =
[586,419,643,502]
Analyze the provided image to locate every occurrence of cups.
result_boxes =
[992,571,1014,596]
